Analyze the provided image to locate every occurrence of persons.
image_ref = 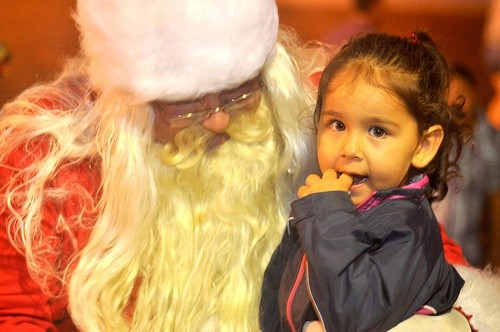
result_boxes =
[433,63,500,269]
[0,1,500,332]
[257,32,466,331]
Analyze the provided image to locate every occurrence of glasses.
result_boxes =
[154,77,265,130]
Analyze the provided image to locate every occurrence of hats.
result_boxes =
[75,0,280,109]
